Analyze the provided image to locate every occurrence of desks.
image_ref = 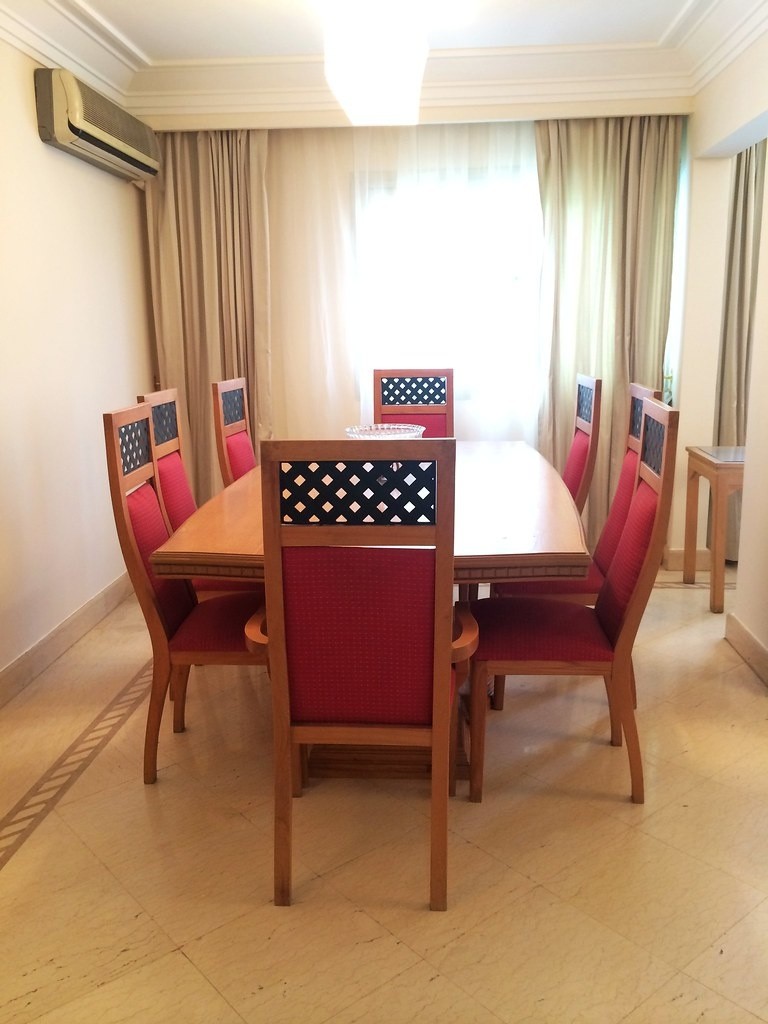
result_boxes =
[147,438,597,787]
[682,446,748,615]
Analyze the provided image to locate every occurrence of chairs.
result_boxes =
[242,438,478,913]
[455,397,682,806]
[489,379,662,711]
[372,369,453,438]
[136,387,265,701]
[212,377,256,491]
[104,403,272,787]
[468,372,602,603]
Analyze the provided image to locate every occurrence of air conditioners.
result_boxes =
[33,67,163,182]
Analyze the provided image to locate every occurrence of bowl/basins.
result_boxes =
[344,423,426,441]
